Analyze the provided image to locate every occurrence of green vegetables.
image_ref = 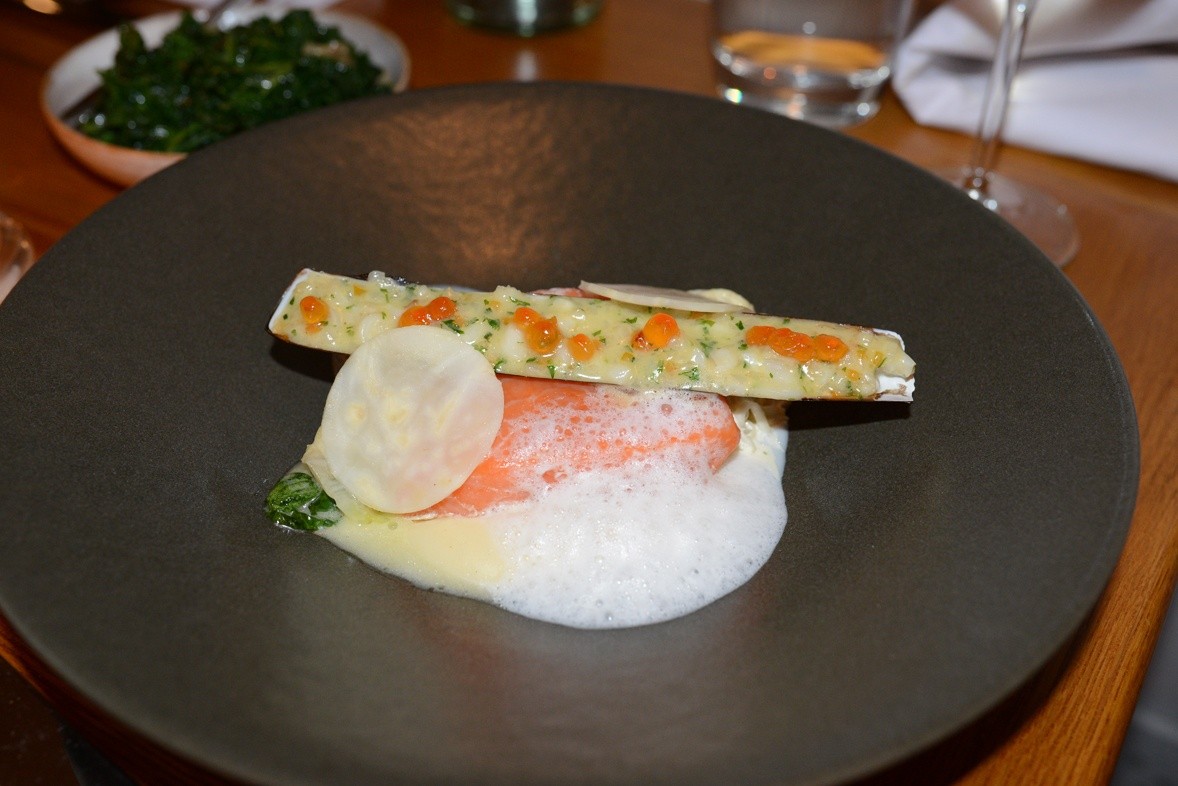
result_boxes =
[55,1,398,151]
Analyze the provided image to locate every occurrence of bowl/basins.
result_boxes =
[36,4,418,188]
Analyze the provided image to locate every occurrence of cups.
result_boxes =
[709,0,919,124]
[448,0,605,35]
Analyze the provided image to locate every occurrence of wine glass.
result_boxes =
[928,1,1081,269]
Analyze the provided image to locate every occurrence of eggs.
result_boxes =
[271,284,792,632]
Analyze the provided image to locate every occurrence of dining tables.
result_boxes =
[0,4,1178,786]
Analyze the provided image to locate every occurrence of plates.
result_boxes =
[6,74,1138,786]
